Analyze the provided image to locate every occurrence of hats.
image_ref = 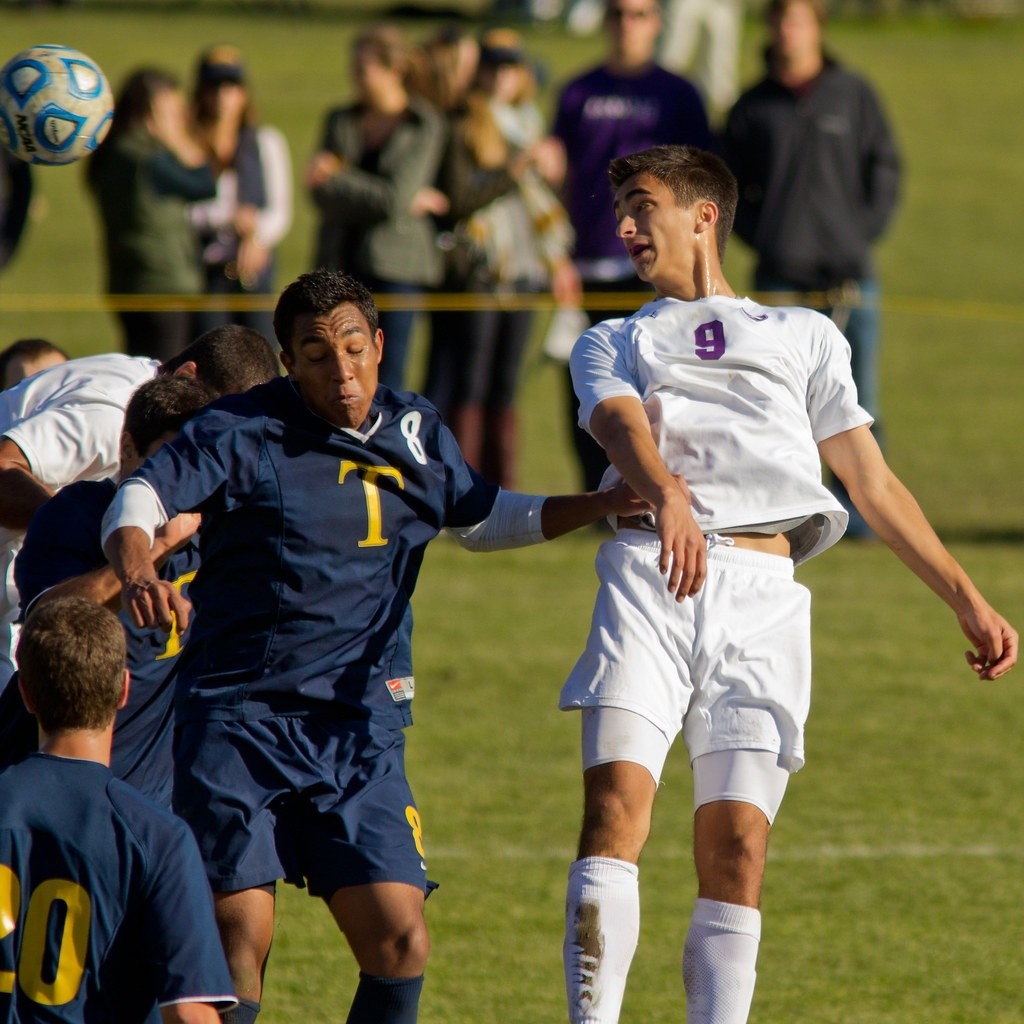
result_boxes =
[197,47,244,84]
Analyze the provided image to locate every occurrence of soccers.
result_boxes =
[0,43,114,166]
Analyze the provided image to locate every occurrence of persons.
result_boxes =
[1,270,691,1024]
[564,147,1019,1024]
[311,1,903,540]
[81,47,295,368]
